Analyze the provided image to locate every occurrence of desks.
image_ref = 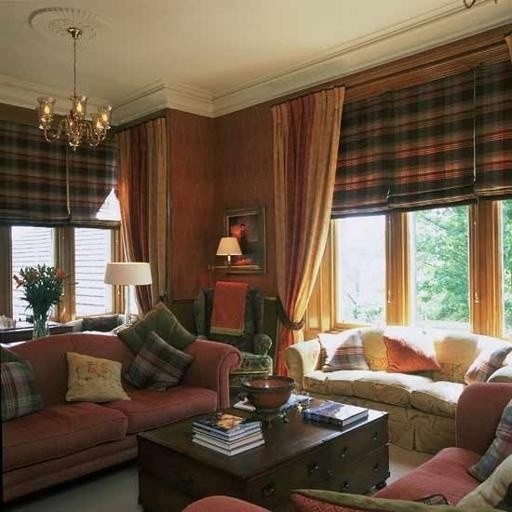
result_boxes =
[0,317,75,344]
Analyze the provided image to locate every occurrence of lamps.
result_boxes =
[216,237,243,267]
[35,28,115,152]
[103,261,153,329]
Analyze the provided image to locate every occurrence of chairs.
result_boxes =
[65,313,138,333]
[201,288,275,390]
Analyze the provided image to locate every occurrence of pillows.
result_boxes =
[318,330,371,371]
[1,360,47,422]
[288,488,506,512]
[1,345,21,362]
[464,340,512,385]
[462,394,511,481]
[454,453,511,509]
[124,331,197,392]
[82,314,120,332]
[64,350,133,403]
[383,328,442,373]
[118,302,199,356]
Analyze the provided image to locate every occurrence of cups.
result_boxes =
[294,392,312,410]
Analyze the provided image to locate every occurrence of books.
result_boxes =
[304,399,369,429]
[191,411,267,455]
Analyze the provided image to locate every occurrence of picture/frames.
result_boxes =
[220,203,268,274]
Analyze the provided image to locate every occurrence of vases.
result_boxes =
[32,310,51,339]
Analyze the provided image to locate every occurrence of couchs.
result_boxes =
[0,336,243,503]
[285,323,511,456]
[180,381,510,512]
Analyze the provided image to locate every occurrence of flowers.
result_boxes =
[11,262,80,334]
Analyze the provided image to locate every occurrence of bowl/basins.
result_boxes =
[240,377,295,408]
